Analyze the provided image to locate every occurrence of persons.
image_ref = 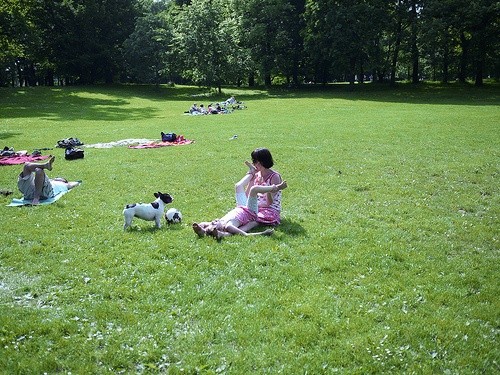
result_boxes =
[189,93,242,116]
[18,155,80,207]
[192,148,282,241]
[205,159,288,238]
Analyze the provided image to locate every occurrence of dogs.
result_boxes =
[122,191,174,231]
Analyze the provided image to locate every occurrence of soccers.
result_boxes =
[165,208,182,225]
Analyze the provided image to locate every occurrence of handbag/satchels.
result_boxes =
[64,146,85,160]
